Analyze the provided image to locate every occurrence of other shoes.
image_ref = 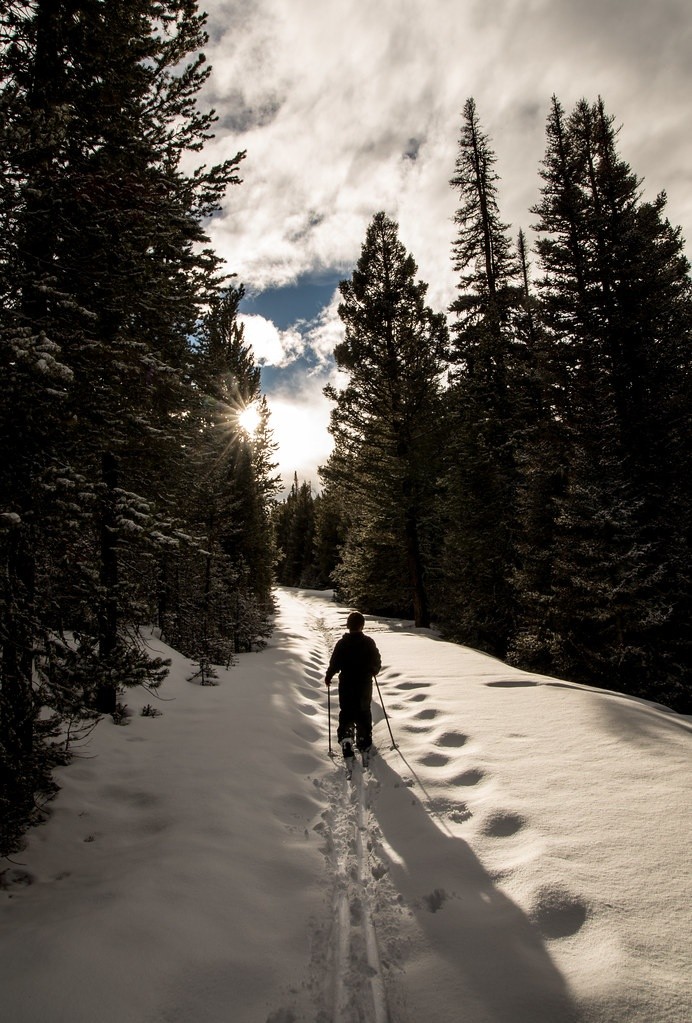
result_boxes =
[356,738,372,753]
[340,738,354,758]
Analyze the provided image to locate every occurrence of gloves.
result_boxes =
[325,673,332,686]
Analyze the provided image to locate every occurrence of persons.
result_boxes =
[325,613,381,760]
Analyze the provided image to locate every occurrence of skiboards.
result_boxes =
[346,754,369,782]
